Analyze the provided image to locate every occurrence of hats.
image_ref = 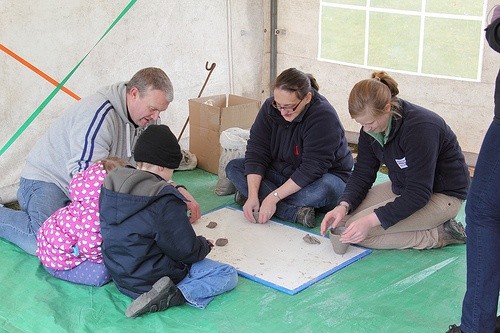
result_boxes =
[134,125,182,168]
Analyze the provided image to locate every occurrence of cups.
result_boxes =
[329,226,350,255]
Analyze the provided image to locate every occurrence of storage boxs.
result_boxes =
[188,93,261,175]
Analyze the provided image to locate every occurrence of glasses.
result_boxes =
[271,99,303,112]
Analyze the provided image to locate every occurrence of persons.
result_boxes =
[99,125,238,318]
[1,67,200,258]
[321,72,469,249]
[224,68,353,229]
[445,4,500,333]
[36,158,126,287]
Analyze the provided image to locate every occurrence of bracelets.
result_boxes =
[339,203,348,215]
[176,185,187,190]
[273,192,281,200]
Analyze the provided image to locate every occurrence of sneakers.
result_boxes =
[235,190,246,207]
[125,276,185,319]
[437,218,467,246]
[296,206,315,228]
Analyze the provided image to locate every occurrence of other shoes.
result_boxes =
[446,324,463,333]
[495,316,500,333]
